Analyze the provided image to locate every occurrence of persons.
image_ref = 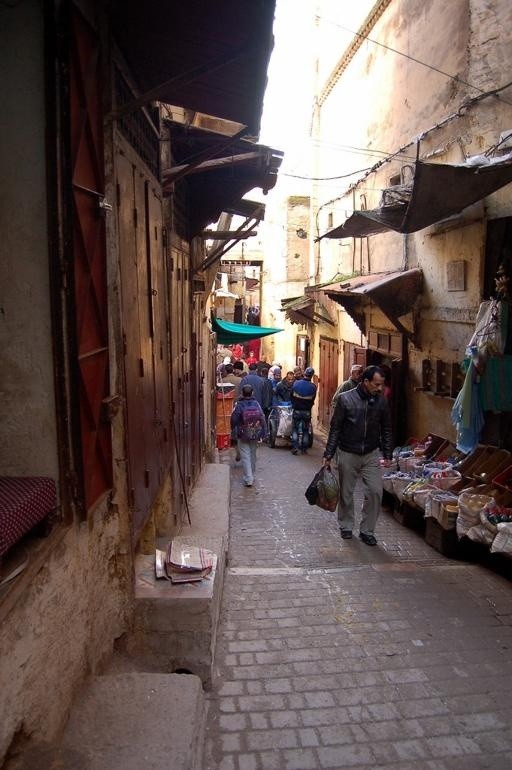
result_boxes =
[217,339,317,488]
[323,365,395,547]
[331,364,363,409]
[247,305,261,325]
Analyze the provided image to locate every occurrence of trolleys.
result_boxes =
[268,394,313,449]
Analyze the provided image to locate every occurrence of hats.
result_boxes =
[232,361,243,371]
[350,365,364,372]
[305,367,315,376]
[273,368,281,375]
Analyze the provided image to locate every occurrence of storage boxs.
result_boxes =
[216,382,235,398]
[393,432,512,554]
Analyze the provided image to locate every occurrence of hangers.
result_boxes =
[475,306,503,337]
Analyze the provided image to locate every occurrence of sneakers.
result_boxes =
[242,476,255,487]
[341,530,353,539]
[358,531,378,546]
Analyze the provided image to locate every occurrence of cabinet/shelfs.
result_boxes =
[216,398,234,447]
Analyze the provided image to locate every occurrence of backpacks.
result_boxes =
[237,401,266,442]
